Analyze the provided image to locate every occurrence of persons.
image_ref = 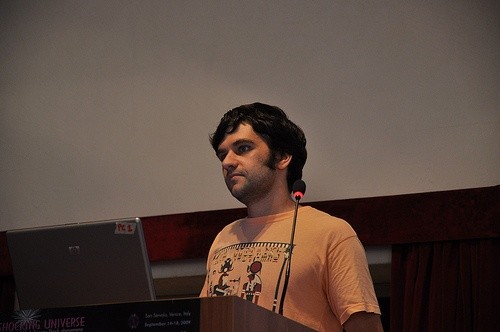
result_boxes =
[191,100,385,332]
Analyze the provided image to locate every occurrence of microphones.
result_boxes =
[279,180,307,317]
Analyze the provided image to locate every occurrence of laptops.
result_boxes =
[6,218,156,311]
